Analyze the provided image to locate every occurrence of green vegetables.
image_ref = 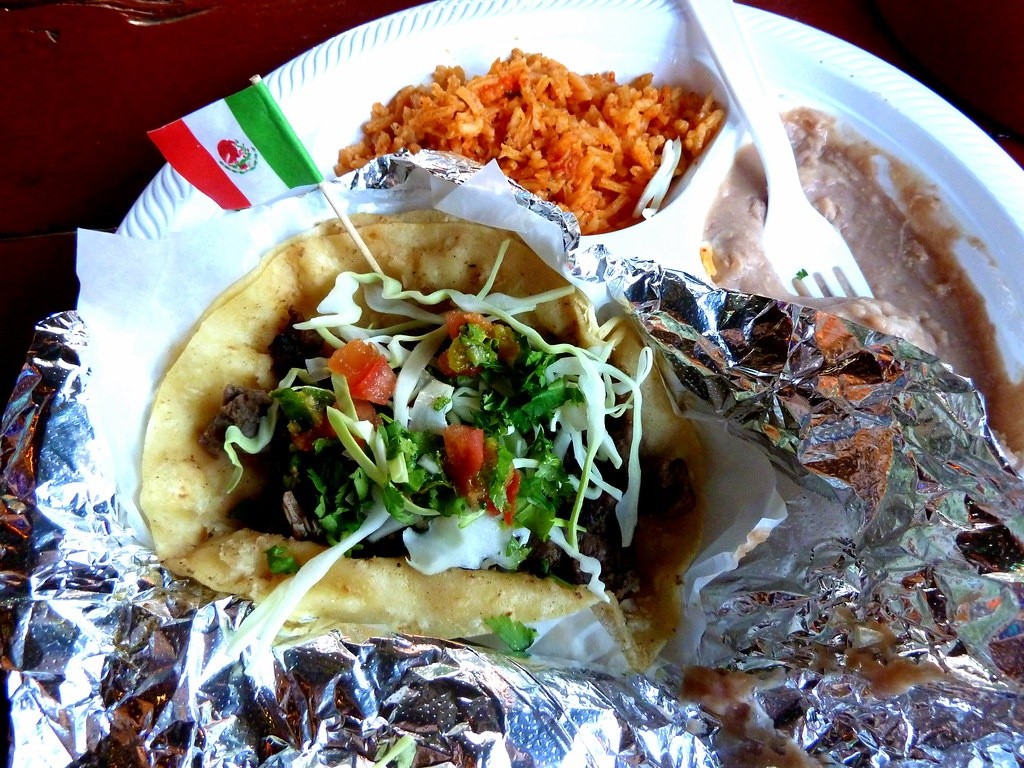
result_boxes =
[264,321,585,658]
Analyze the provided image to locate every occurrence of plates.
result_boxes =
[116,0,1020,471]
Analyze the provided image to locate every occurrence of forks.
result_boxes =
[680,0,873,302]
